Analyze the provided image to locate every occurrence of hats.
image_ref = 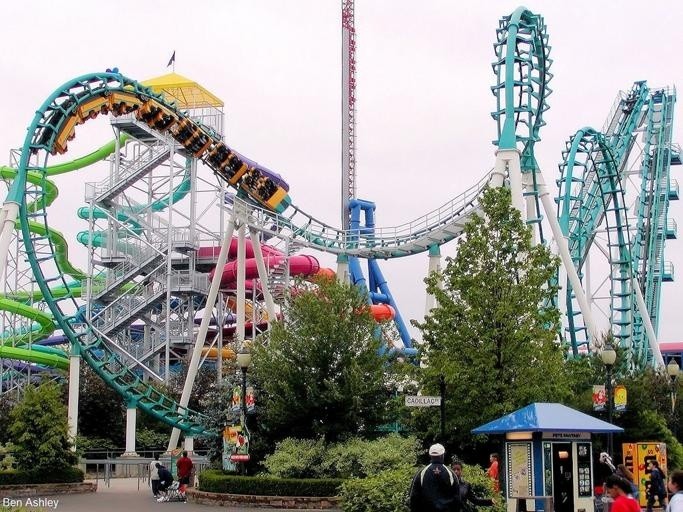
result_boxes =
[429,443,445,456]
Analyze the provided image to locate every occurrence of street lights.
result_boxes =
[236,347,251,475]
[602,344,617,470]
[667,356,679,436]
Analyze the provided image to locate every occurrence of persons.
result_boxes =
[646,461,667,512]
[666,469,683,512]
[176,450,193,488]
[407,443,461,512]
[617,464,634,481]
[487,452,499,480]
[606,474,642,512]
[451,462,498,512]
[156,463,174,490]
[150,454,162,497]
[117,102,277,201]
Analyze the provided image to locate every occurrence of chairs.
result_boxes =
[162,480,185,503]
